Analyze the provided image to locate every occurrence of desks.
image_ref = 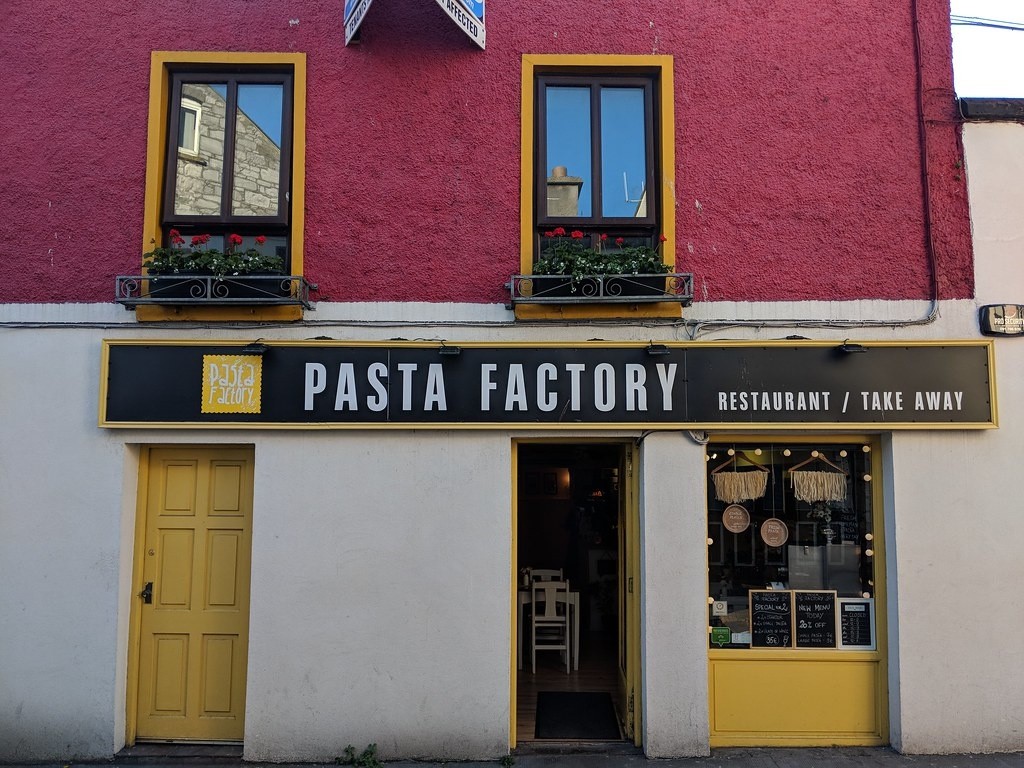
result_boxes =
[518,591,579,671]
[721,595,748,613]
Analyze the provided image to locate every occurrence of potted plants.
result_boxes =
[581,578,617,658]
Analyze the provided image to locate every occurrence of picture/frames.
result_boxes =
[734,523,755,567]
[763,544,786,566]
[708,521,725,566]
[795,522,818,566]
[829,522,847,565]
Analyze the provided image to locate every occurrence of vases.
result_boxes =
[147,269,292,307]
[533,270,667,296]
[523,574,529,585]
[722,586,727,597]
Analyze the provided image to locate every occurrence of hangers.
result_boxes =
[711,445,770,475]
[787,446,849,476]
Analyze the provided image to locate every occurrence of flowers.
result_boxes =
[532,227,676,292]
[519,567,532,574]
[720,575,728,586]
[141,229,286,283]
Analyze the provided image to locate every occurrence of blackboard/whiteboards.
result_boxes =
[792,590,839,650]
[748,589,794,650]
[836,597,878,652]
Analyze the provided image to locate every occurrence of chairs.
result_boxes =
[529,569,570,675]
